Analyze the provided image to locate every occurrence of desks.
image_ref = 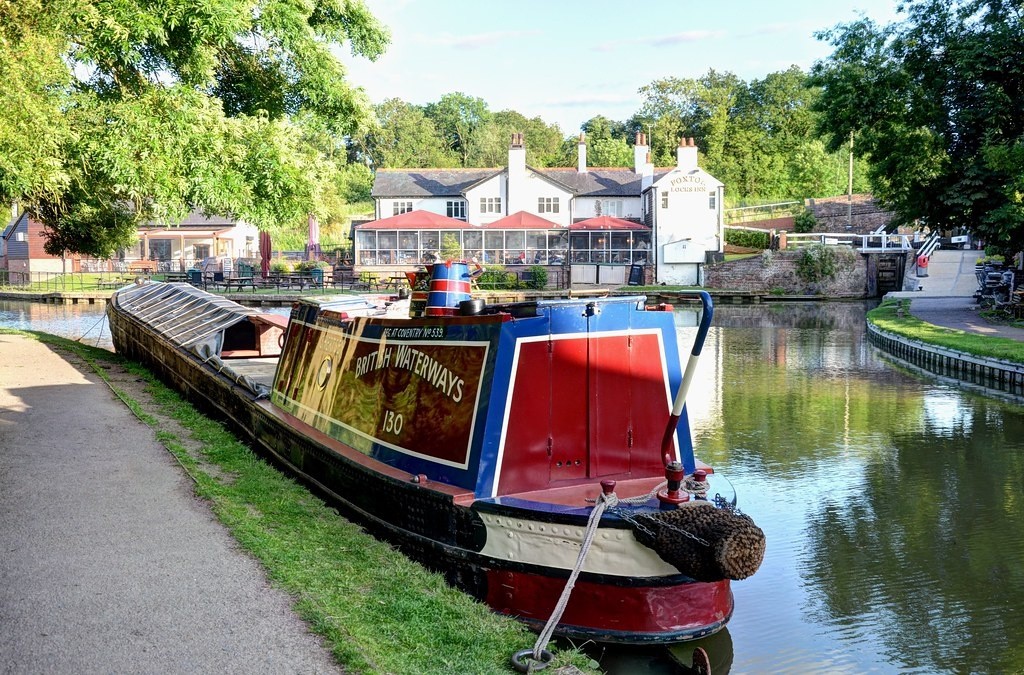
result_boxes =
[226,276,339,293]
[350,277,380,290]
[386,276,408,290]
[122,277,150,288]
[130,267,153,276]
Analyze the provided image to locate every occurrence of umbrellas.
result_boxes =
[258,228,272,279]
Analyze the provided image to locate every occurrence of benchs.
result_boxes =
[220,281,340,287]
[96,281,132,289]
[128,271,155,274]
[163,273,190,283]
[345,281,407,287]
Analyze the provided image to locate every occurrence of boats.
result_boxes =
[106,257,765,653]
